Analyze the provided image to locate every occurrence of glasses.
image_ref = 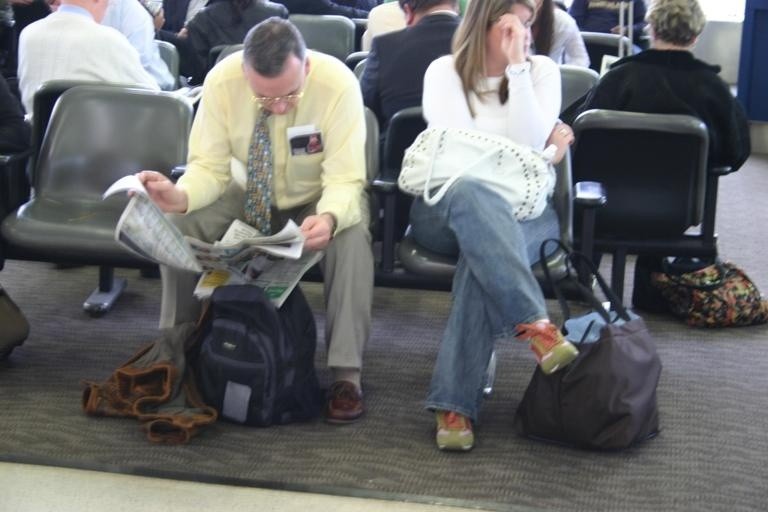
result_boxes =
[251,89,304,103]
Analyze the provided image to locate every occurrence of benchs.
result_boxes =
[0,0,734,389]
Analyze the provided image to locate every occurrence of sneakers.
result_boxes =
[435,408,475,453]
[514,322,580,380]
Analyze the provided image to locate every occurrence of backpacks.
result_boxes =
[198,282,330,428]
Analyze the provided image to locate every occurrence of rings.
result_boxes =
[560,128,569,136]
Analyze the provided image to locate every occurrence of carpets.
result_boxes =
[0,150,768,510]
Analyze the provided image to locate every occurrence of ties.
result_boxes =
[244,106,273,237]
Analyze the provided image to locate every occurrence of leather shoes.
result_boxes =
[324,380,366,424]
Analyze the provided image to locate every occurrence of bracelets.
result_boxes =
[506,63,529,76]
[329,209,336,240]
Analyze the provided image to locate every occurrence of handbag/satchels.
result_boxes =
[649,255,768,327]
[397,127,557,222]
[513,309,663,453]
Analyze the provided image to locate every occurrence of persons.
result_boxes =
[405,1,580,448]
[584,2,753,247]
[143,0,462,179]
[126,15,377,425]
[0,0,177,149]
[517,2,589,71]
[609,23,649,36]
